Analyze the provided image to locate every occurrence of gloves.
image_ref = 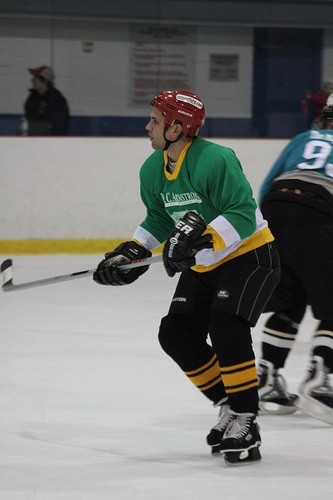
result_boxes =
[93,241,152,286]
[162,211,214,277]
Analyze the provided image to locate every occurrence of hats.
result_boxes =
[28,67,54,85]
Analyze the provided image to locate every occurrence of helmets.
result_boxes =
[322,94,333,119]
[151,90,206,136]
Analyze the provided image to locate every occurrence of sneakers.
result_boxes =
[256,359,299,414]
[294,355,333,427]
[206,399,237,456]
[220,407,262,466]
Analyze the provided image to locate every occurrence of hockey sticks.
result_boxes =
[0,255,163,292]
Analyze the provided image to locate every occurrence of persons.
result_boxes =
[93,91,279,465]
[301,81,333,118]
[23,66,68,137]
[258,93,333,425]
